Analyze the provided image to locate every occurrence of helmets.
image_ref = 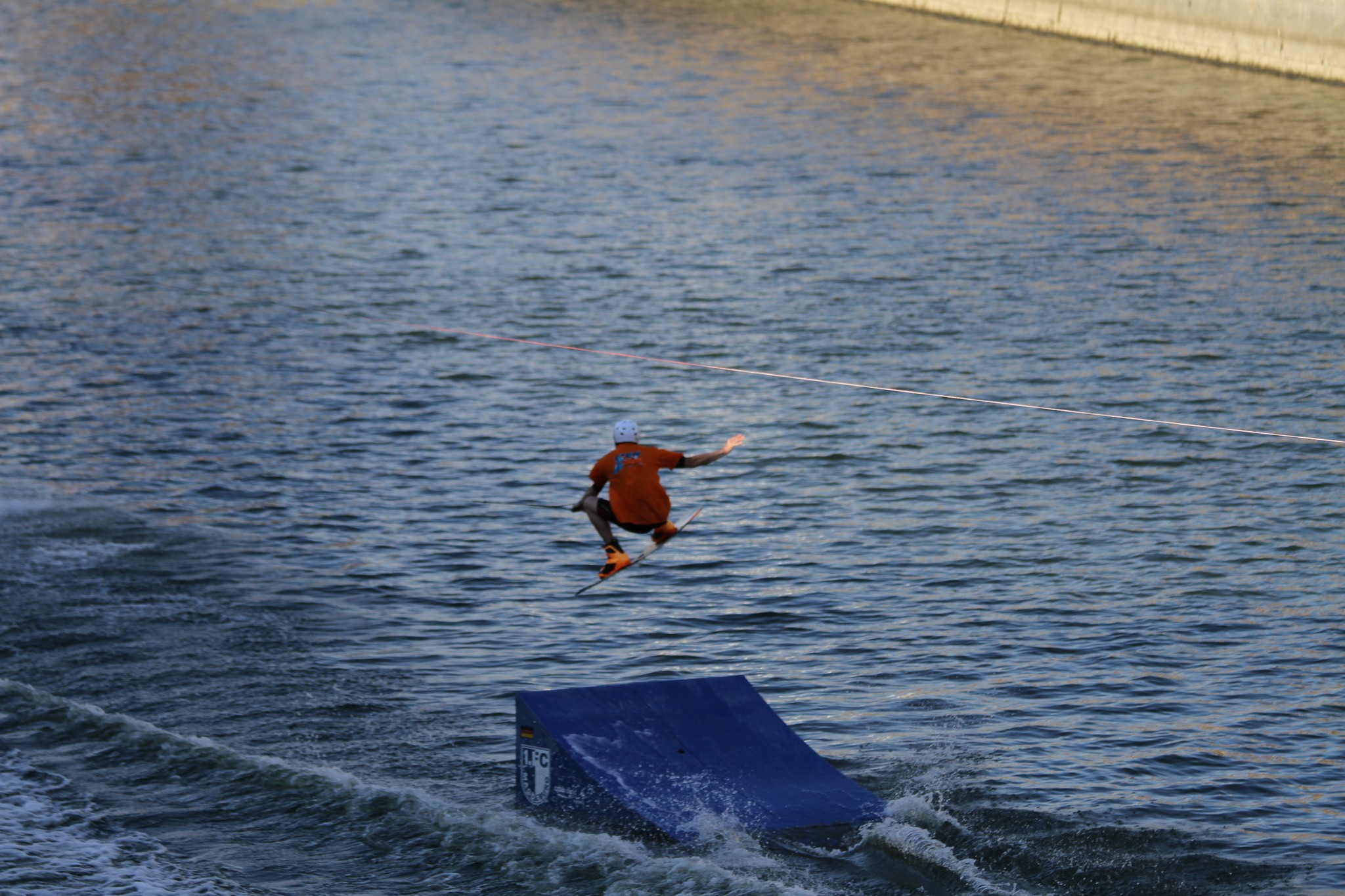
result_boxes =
[612,419,640,445]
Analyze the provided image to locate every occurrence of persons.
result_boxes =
[570,419,744,579]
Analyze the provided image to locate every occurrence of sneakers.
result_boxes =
[652,521,677,542]
[598,538,631,579]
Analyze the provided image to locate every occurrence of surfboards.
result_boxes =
[573,505,709,599]
